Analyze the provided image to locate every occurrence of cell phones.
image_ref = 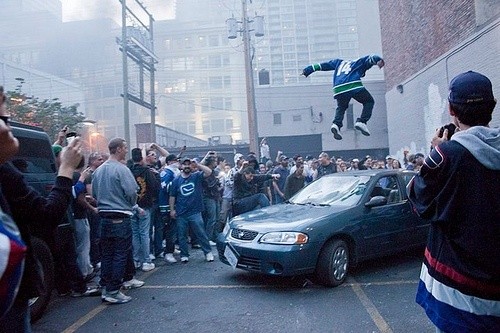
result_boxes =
[65,131,76,145]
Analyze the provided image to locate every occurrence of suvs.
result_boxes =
[4,118,77,323]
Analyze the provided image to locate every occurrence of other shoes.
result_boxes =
[192,244,201,248]
[209,240,216,245]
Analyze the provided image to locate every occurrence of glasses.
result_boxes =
[0,114,11,126]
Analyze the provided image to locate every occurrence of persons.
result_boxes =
[300,55,385,140]
[405,70,500,333]
[0,86,500,333]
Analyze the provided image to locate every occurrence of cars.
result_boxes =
[216,168,432,287]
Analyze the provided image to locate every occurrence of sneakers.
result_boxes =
[181,257,189,262]
[355,122,370,136]
[330,124,342,140]
[59,244,180,303]
[206,251,214,262]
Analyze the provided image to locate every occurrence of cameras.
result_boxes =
[438,123,456,141]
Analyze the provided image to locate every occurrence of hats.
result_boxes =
[386,155,392,160]
[166,154,179,163]
[448,71,493,103]
[179,157,190,165]
[280,155,288,161]
